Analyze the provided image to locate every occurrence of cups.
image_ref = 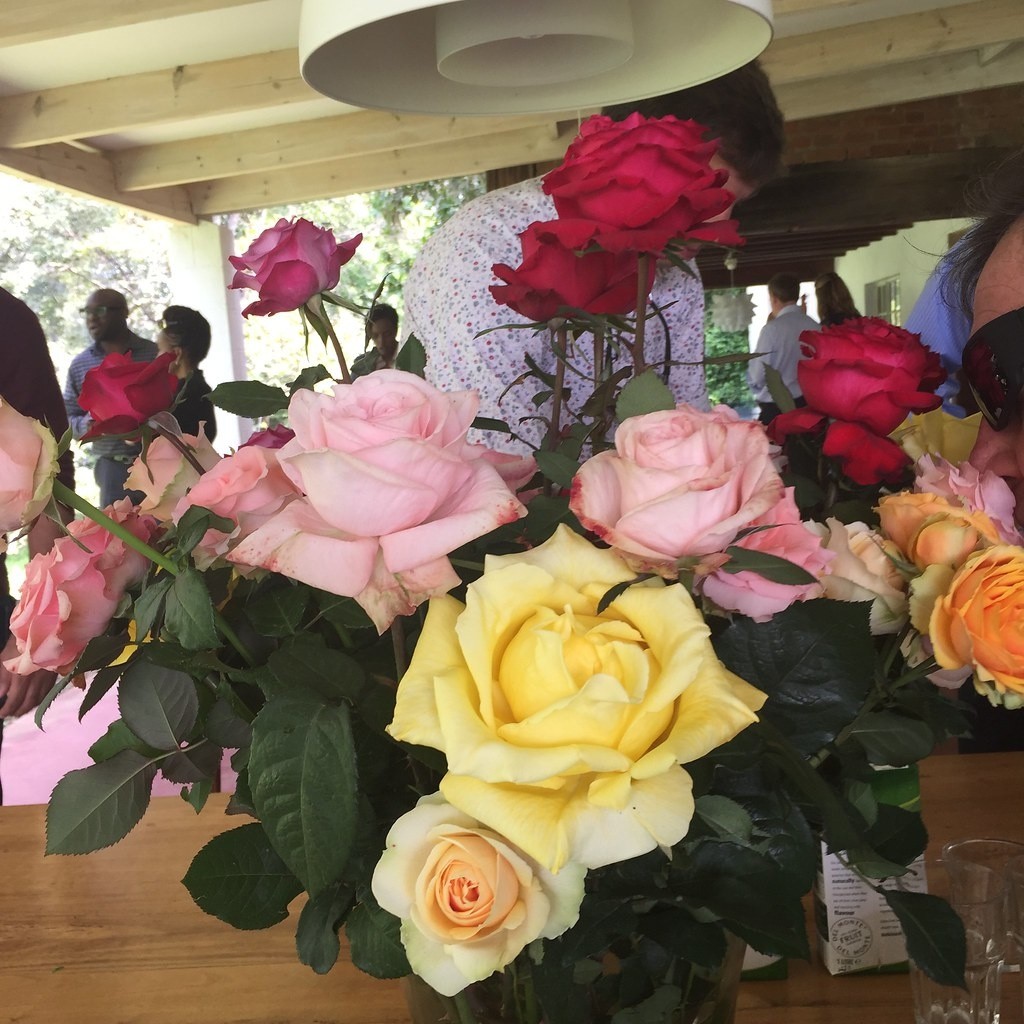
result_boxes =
[938,836,1023,975]
[901,852,1009,1021]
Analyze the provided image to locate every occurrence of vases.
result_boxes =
[404,925,748,1024]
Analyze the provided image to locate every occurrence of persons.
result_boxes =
[0,286,79,807]
[349,303,400,379]
[746,271,823,426]
[814,272,863,327]
[904,152,1024,533]
[61,286,160,511]
[391,49,790,480]
[152,304,218,445]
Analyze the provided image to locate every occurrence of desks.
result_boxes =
[0,747,1021,1023]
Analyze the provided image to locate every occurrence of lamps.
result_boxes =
[301,2,774,113]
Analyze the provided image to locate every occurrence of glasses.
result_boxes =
[952,304,1024,432]
[77,305,125,323]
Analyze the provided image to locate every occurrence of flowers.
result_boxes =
[0,109,1022,1024]
